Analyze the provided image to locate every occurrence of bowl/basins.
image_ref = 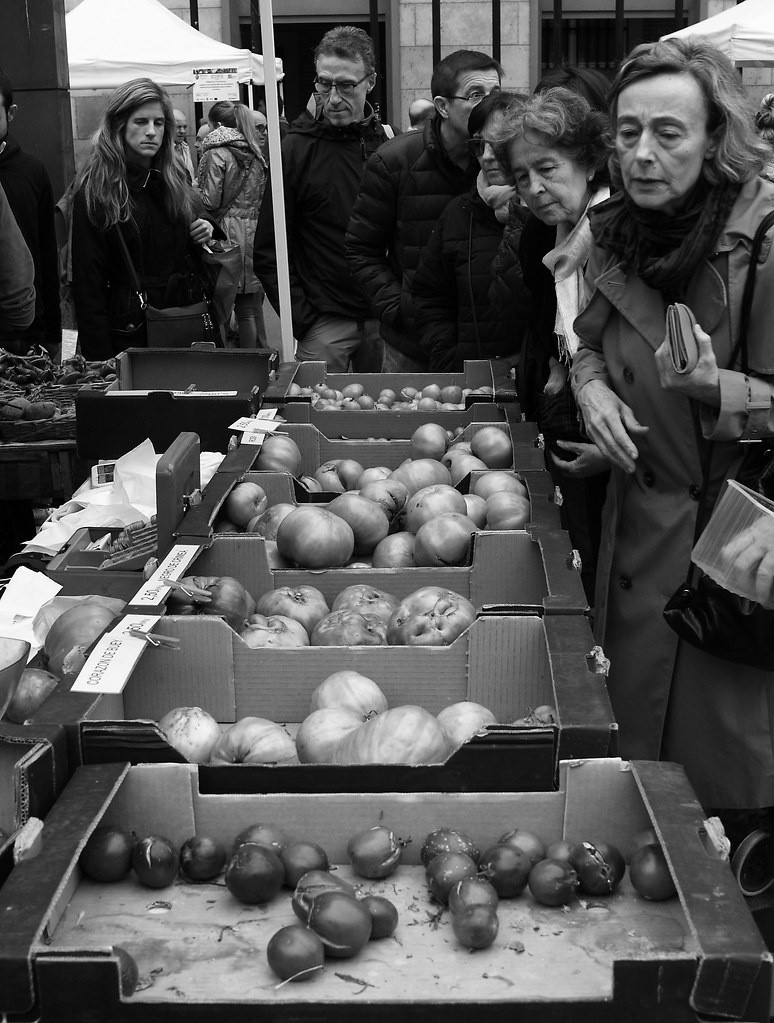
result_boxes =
[692,480,774,612]
[0,637,31,724]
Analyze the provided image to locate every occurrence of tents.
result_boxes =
[661,0,774,68]
[64,0,285,119]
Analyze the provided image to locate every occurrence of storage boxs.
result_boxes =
[0,347,774,1023]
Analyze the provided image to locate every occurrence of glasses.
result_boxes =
[444,94,484,107]
[255,124,267,133]
[313,74,370,97]
[464,138,496,157]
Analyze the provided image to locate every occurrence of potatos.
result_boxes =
[0,398,57,420]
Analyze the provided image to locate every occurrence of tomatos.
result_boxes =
[81,821,677,983]
[289,384,495,410]
[172,576,477,648]
[214,424,530,568]
[158,671,556,766]
[5,601,116,722]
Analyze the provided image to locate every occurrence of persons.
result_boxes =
[755,93,774,141]
[171,102,280,349]
[70,77,227,361]
[533,66,611,113]
[252,26,404,373]
[0,181,36,331]
[413,92,534,373]
[0,69,62,361]
[344,51,502,373]
[569,37,774,808]
[484,87,610,588]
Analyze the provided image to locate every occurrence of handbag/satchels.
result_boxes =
[195,235,244,341]
[663,442,772,674]
[143,300,224,350]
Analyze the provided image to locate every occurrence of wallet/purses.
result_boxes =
[666,302,701,375]
